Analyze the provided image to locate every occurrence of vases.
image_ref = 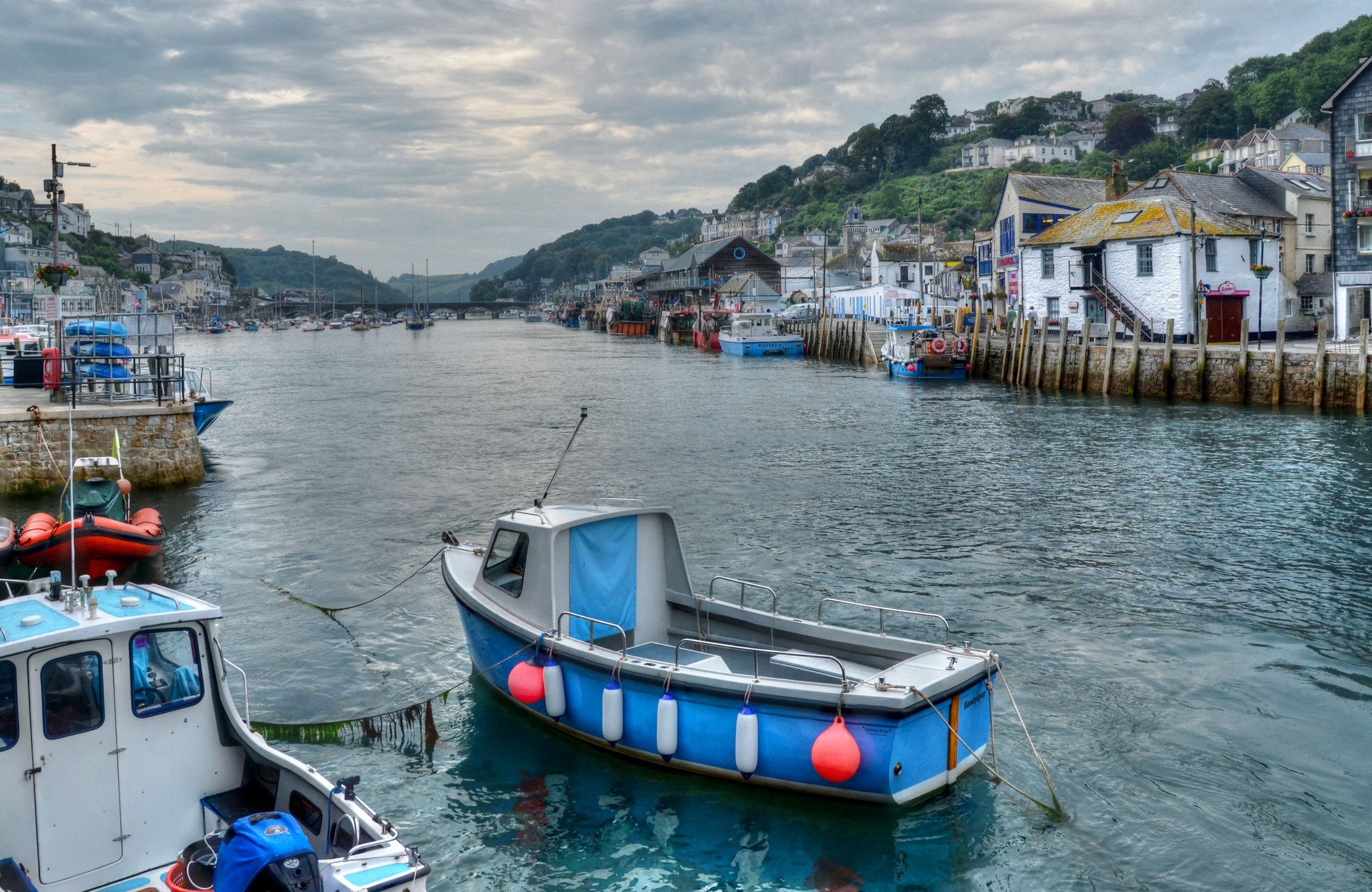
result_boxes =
[1352,212,1357,217]
[1253,270,1271,279]
[1359,213,1364,217]
[1365,212,1371,217]
[41,272,71,287]
[1049,322,1058,326]
[988,296,992,300]
[971,297,977,301]
[998,296,1004,300]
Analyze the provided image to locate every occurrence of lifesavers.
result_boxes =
[703,313,711,321]
[43,355,52,380]
[932,338,947,353]
[952,339,967,354]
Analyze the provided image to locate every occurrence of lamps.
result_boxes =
[880,274,883,283]
[1189,225,1211,252]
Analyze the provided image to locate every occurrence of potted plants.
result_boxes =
[1346,149,1354,158]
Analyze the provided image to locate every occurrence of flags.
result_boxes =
[135,295,141,308]
[112,432,120,459]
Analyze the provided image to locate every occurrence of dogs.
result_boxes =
[990,327,997,339]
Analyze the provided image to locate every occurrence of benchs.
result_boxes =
[1074,322,1109,345]
[768,650,887,685]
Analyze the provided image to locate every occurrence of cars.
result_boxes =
[776,303,822,325]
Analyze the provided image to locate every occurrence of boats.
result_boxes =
[1,572,427,892]
[70,340,132,362]
[74,360,131,383]
[65,320,129,340]
[717,270,804,359]
[0,400,168,585]
[881,320,972,382]
[441,406,1004,814]
[0,319,67,384]
[503,259,741,350]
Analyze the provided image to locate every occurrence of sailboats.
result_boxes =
[172,238,449,335]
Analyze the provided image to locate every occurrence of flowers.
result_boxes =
[1341,207,1372,218]
[968,292,979,300]
[994,292,1008,299]
[34,263,79,289]
[1248,262,1274,271]
[1049,319,1057,323]
[982,292,995,299]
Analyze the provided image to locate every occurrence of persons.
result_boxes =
[15,317,18,325]
[1027,306,1038,338]
[1004,304,1017,338]
[985,308,995,339]
[961,303,971,331]
